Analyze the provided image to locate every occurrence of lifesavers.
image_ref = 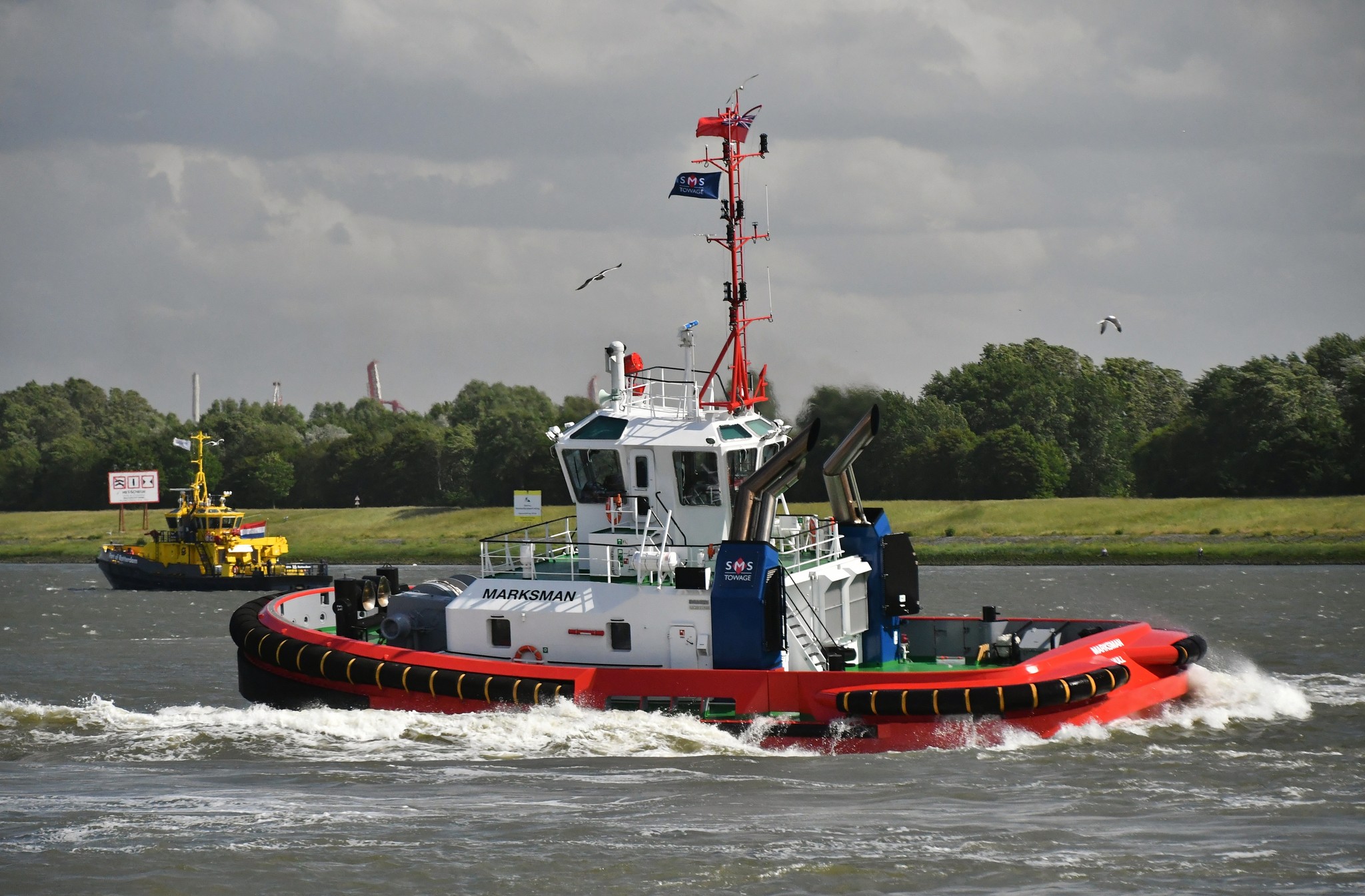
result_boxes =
[809,519,816,549]
[232,566,238,574]
[605,497,622,525]
[514,645,542,665]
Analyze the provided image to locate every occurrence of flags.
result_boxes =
[694,115,756,144]
[240,521,266,539]
[668,171,723,201]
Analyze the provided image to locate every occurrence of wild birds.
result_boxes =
[575,262,624,292]
[202,438,225,447]
[1096,314,1124,335]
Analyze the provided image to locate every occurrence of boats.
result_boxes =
[230,71,1207,754]
[94,430,333,592]
